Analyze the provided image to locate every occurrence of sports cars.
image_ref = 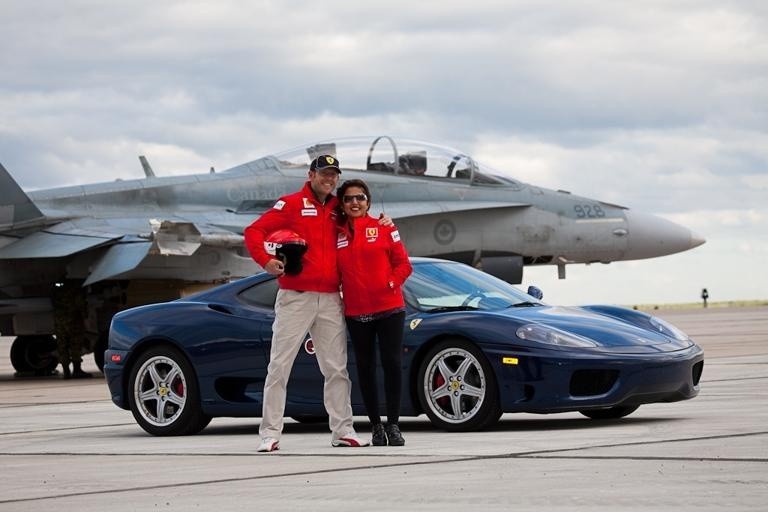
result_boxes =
[104,255,704,437]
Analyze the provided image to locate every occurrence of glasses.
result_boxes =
[343,194,369,203]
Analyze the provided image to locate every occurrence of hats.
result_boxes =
[310,155,342,175]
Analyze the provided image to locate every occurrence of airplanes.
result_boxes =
[0,134,710,379]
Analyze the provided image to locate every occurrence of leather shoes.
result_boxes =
[386,424,405,446]
[372,424,387,446]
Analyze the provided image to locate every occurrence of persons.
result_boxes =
[244,155,394,452]
[50,268,91,378]
[337,179,413,446]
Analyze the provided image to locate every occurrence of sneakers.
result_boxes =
[332,434,370,447]
[257,437,280,452]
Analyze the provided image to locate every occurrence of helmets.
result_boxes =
[264,229,307,276]
